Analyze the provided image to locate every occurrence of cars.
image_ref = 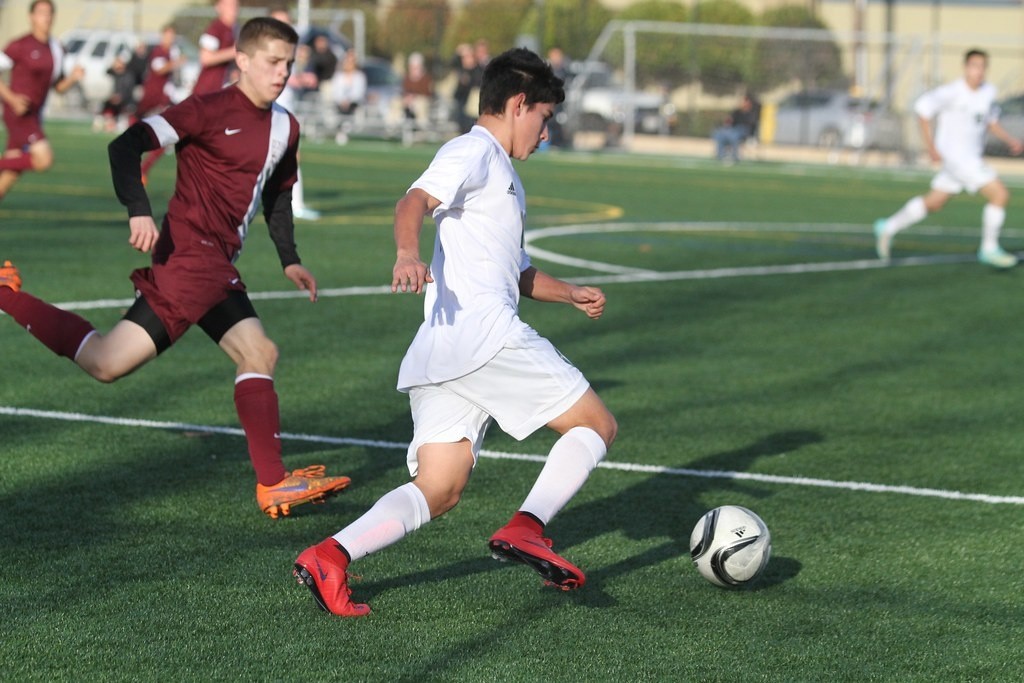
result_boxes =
[55,26,203,112]
[984,93,1024,158]
[291,26,406,121]
[771,88,905,152]
[548,70,676,135]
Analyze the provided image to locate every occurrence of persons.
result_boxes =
[293,48,618,615]
[0,0,567,221]
[0,17,351,519]
[714,93,760,168]
[872,47,1024,267]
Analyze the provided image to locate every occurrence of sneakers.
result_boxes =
[292,546,370,616]
[0,261,23,292]
[488,523,585,590]
[256,465,351,518]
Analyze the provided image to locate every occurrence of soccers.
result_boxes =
[688,505,772,589]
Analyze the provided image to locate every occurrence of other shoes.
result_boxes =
[872,217,891,261]
[291,208,318,220]
[142,172,147,186]
[977,244,1018,267]
